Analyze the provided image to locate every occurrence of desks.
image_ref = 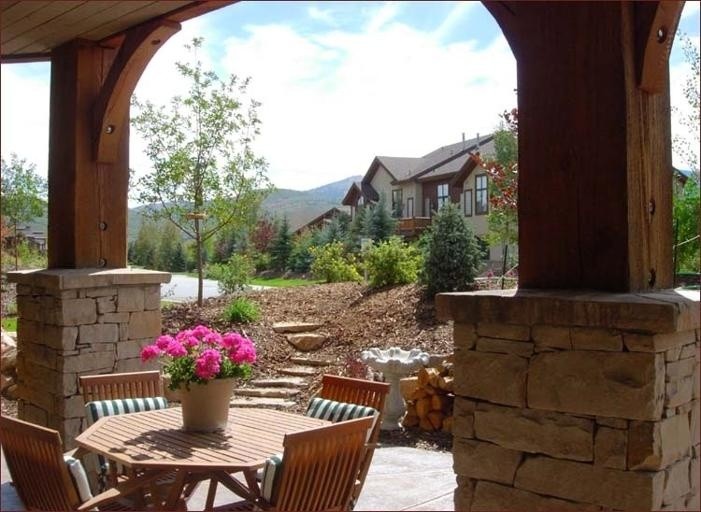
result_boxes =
[73,405,333,512]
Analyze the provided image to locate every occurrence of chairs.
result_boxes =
[78,369,171,506]
[0,412,165,512]
[205,415,374,512]
[256,373,391,512]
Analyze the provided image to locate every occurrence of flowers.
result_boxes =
[140,324,257,393]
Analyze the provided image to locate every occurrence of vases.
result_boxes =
[179,378,235,429]
